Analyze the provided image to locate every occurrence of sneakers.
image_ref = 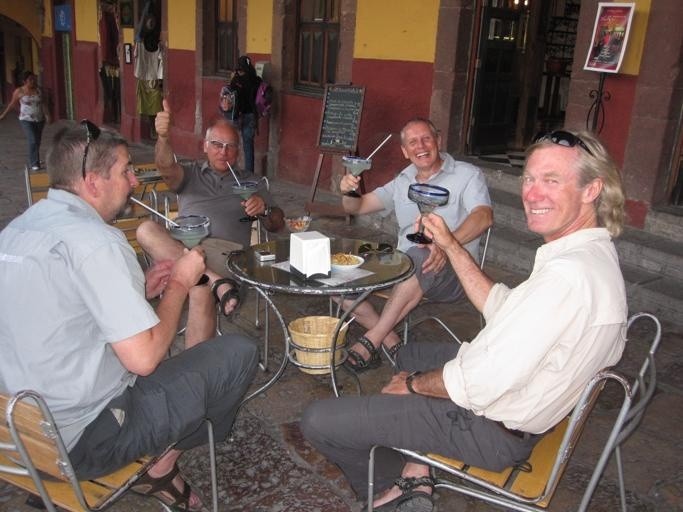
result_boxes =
[32,166,39,170]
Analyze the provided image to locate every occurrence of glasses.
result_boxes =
[80,118,101,181]
[531,131,594,155]
[208,140,239,150]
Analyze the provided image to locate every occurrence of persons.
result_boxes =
[136,99,288,350]
[0,72,52,170]
[331,117,494,373]
[298,127,632,512]
[218,54,274,177]
[0,119,262,511]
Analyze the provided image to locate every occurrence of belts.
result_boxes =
[495,422,537,439]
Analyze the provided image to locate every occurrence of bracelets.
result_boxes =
[260,202,272,217]
[403,371,421,393]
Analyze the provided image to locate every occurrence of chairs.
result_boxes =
[366,313,663,512]
[0,390,219,512]
[328,228,494,371]
[23,157,224,337]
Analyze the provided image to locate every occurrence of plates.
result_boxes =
[330,254,365,270]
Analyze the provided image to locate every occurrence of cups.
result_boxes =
[283,215,311,233]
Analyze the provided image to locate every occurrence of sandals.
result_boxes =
[210,278,249,322]
[129,463,203,512]
[360,475,435,512]
[343,336,403,374]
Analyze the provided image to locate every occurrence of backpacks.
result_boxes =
[218,84,239,124]
[254,81,272,136]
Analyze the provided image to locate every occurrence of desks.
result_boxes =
[226,236,416,442]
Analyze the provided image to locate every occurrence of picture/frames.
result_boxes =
[124,43,132,65]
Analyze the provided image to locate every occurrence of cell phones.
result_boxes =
[255,249,275,262]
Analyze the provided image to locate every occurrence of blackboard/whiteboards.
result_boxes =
[316,84,366,152]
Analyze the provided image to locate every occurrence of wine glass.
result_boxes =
[341,155,372,199]
[405,183,449,244]
[230,181,258,223]
[168,215,210,285]
